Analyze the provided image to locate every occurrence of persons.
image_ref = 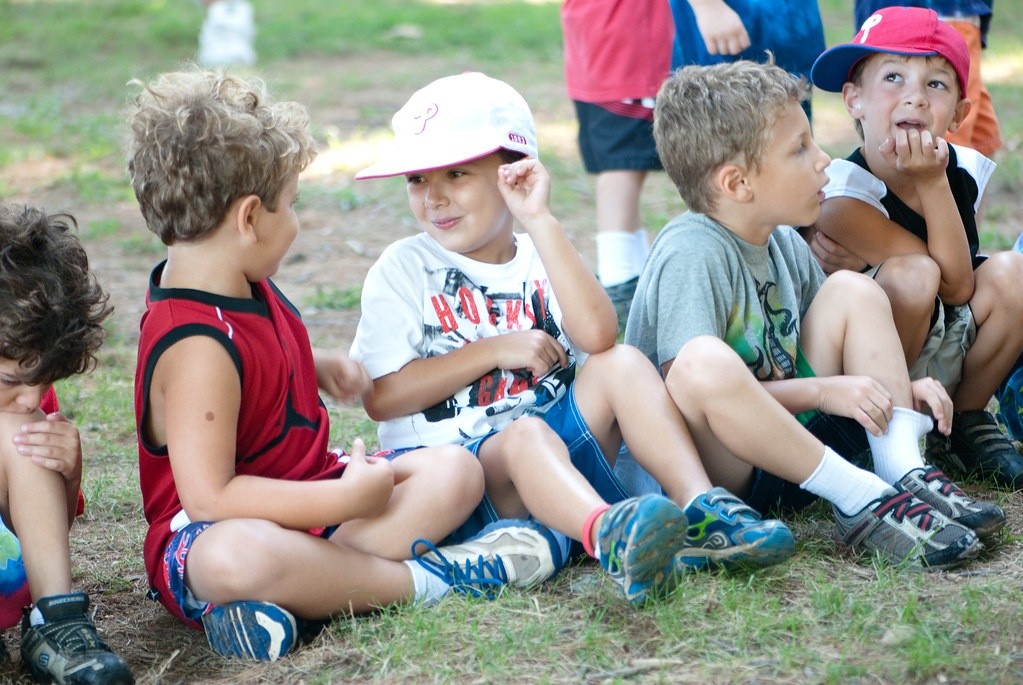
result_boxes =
[560,0,825,331]
[351,73,796,608]
[800,5,1023,494]
[127,73,570,663]
[856,0,1003,213]
[0,204,137,685]
[624,62,1005,567]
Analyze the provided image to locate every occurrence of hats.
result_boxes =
[810,6,971,99]
[354,72,537,181]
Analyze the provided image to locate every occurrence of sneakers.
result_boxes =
[200,600,298,662]
[20,593,136,685]
[932,409,1022,490]
[830,483,979,570]
[678,487,794,572]
[594,493,689,611]
[412,518,562,605]
[893,461,1006,536]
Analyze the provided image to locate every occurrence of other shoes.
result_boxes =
[595,274,640,323]
